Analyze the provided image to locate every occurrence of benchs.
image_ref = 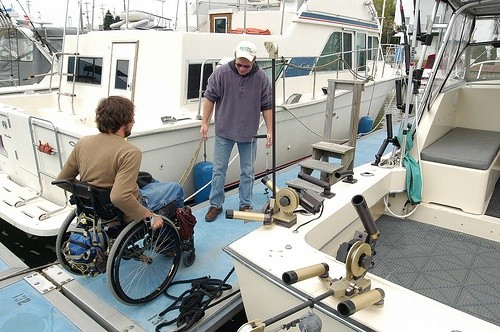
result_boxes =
[420,126,500,217]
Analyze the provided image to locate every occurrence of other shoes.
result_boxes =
[239,206,252,211]
[205,206,223,222]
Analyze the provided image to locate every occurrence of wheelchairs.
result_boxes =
[50,171,198,306]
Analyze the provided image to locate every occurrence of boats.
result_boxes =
[1,0,407,239]
[223,1,500,332]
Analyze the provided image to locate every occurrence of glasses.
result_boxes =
[235,61,253,69]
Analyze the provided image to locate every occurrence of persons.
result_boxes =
[57,96,193,255]
[200,41,274,221]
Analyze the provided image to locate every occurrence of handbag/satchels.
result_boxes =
[61,216,110,280]
[176,205,197,240]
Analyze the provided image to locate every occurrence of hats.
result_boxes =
[235,41,258,62]
